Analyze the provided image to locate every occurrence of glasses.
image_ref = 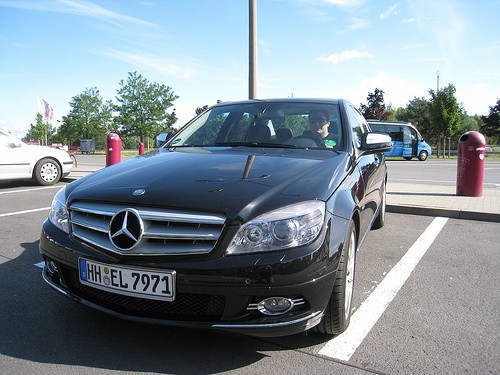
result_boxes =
[309,117,328,123]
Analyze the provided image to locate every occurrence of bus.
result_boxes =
[366,119,431,161]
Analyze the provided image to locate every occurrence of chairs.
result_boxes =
[276,128,292,141]
[247,125,271,143]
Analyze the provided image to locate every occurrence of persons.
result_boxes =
[308,109,338,143]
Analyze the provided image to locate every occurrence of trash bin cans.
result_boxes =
[456,131,487,197]
[106,133,121,166]
[139,142,144,155]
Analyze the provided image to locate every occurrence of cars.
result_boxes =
[0,129,74,186]
[38,97,393,337]
